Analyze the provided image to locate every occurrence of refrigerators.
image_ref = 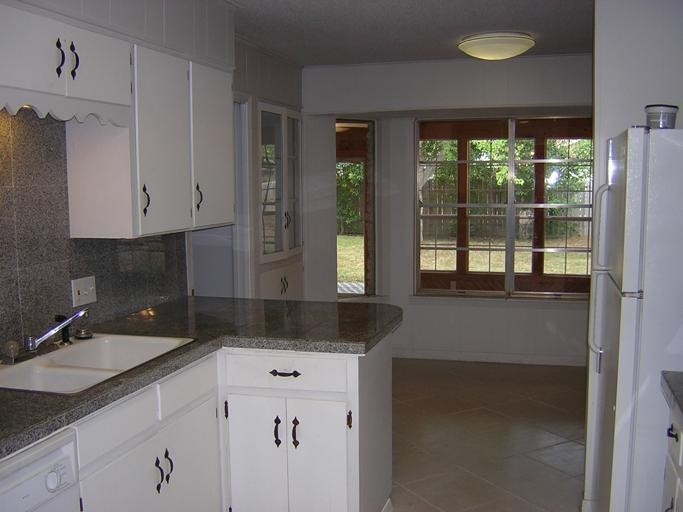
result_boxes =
[587,123,682,510]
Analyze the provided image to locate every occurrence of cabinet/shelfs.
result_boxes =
[186,94,304,301]
[0,3,133,132]
[224,329,396,511]
[73,349,223,511]
[63,40,238,241]
[658,412,682,512]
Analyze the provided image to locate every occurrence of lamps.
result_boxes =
[454,30,535,61]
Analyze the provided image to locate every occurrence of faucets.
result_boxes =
[26,306,90,352]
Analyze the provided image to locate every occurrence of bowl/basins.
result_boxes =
[643,103,678,128]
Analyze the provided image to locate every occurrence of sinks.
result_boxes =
[33,338,184,372]
[4,366,94,391]
